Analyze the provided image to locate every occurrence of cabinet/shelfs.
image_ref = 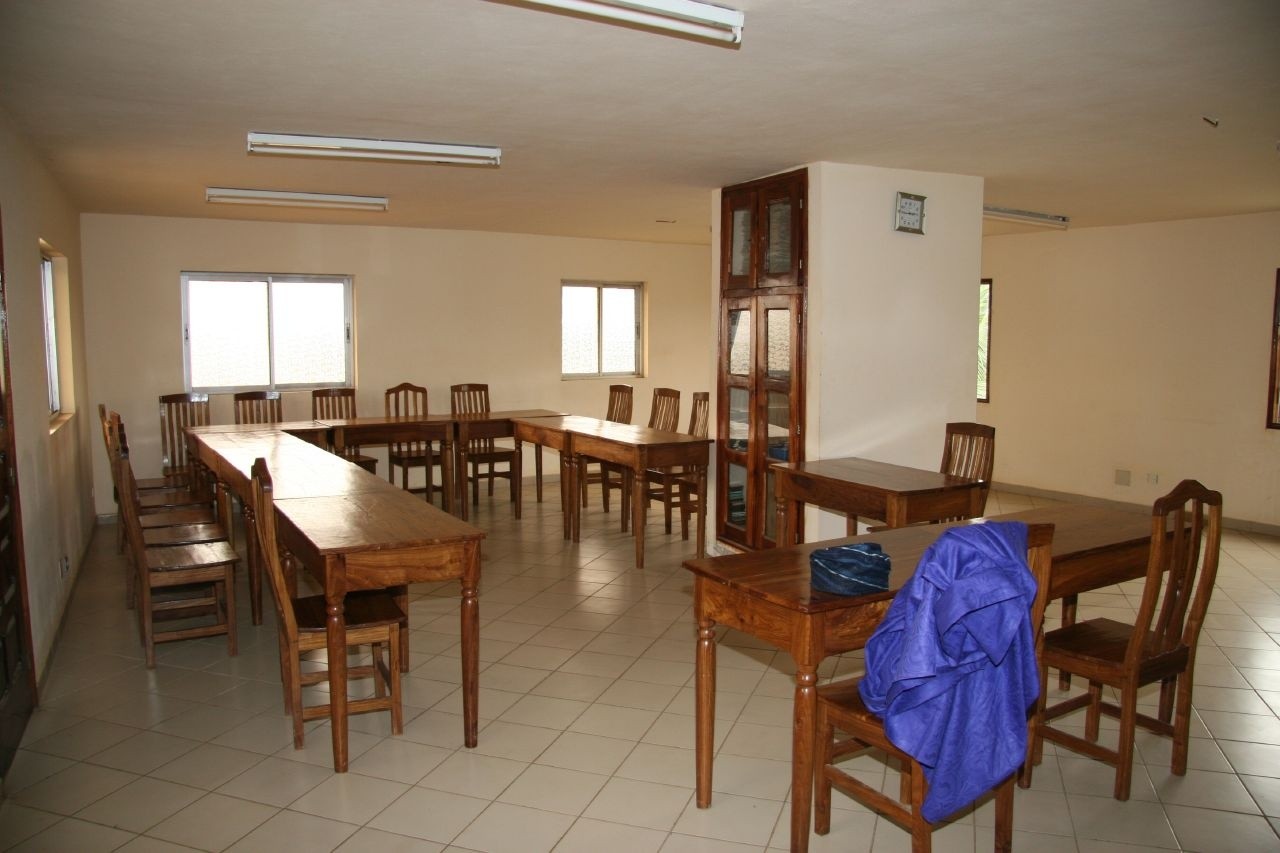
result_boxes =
[705,162,810,556]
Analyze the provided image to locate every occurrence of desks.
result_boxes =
[682,501,1204,852]
[193,407,715,772]
[770,456,987,551]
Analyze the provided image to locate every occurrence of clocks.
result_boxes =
[893,190,928,236]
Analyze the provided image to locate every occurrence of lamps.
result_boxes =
[983,207,1070,230]
[247,131,502,168]
[205,187,390,212]
[517,0,746,44]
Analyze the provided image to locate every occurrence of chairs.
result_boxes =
[385,381,443,507]
[559,385,634,511]
[309,387,378,475]
[248,458,407,750]
[238,388,283,424]
[645,393,710,539]
[816,521,1055,852]
[623,388,682,532]
[448,384,515,506]
[93,394,242,671]
[935,422,999,522]
[1034,480,1224,801]
